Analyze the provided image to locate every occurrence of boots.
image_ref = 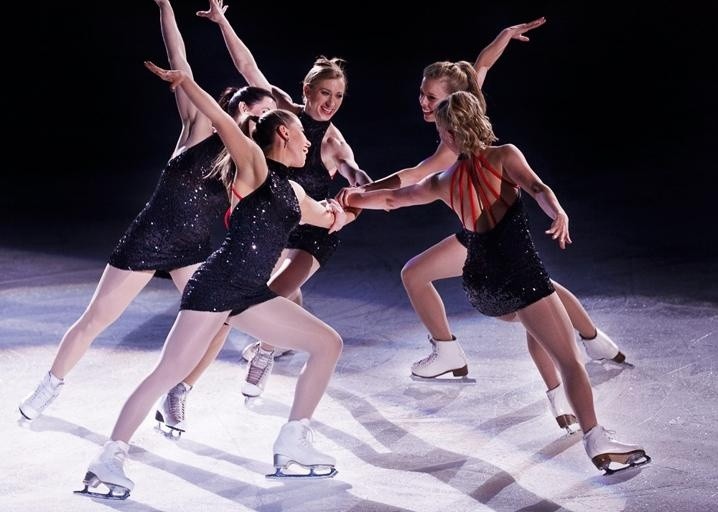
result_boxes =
[19,371,64,420]
[546,384,580,428]
[583,424,646,470]
[84,439,135,491]
[155,381,192,432]
[274,419,336,469]
[242,341,292,397]
[579,327,625,363]
[411,334,468,379]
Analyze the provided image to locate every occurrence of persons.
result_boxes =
[348,92,646,468]
[17,1,345,434]
[82,59,365,493]
[337,15,625,379]
[197,0,373,398]
